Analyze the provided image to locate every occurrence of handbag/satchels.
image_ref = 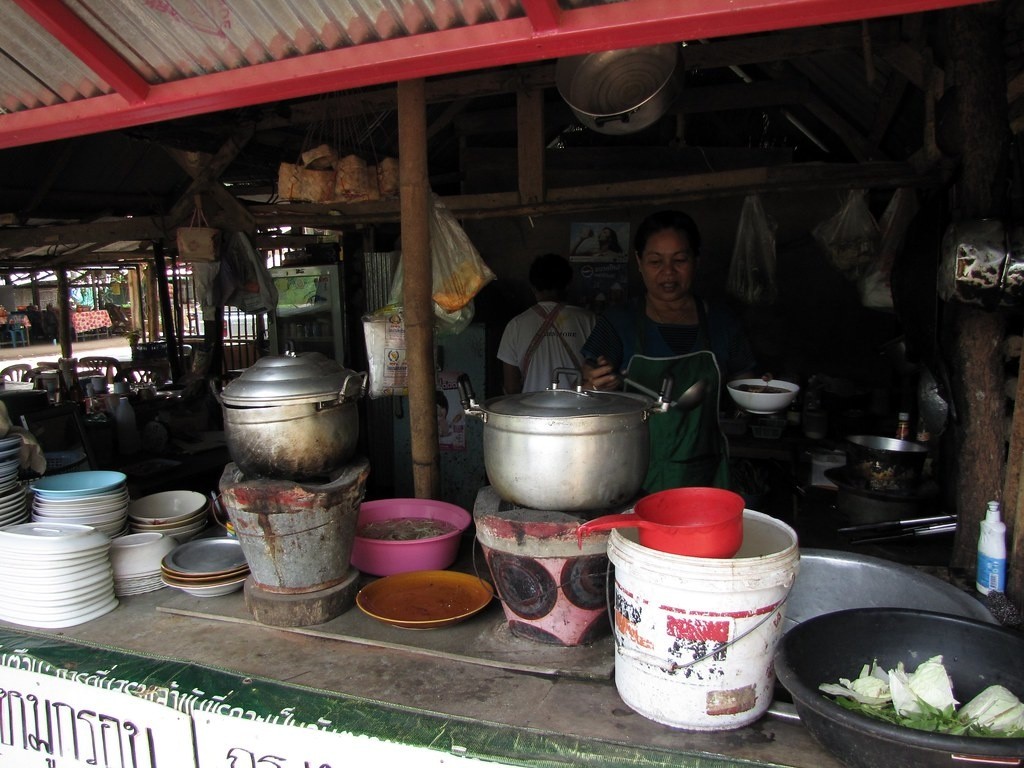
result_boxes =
[813,189,880,281]
[429,186,498,313]
[177,207,222,263]
[360,301,442,400]
[388,251,475,338]
[226,232,279,314]
[722,193,783,316]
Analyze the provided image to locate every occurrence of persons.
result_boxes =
[435,391,453,436]
[498,252,594,395]
[570,228,625,254]
[28,302,61,341]
[580,210,774,493]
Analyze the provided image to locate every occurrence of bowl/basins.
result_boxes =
[726,379,801,415]
[349,498,471,577]
[774,607,1024,768]
[161,579,246,597]
[127,490,212,543]
[110,531,178,596]
[783,546,998,639]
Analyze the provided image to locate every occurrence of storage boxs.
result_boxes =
[719,418,787,438]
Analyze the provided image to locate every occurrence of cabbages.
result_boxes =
[818,655,1024,732]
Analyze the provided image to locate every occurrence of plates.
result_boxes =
[161,537,250,585]
[0,436,131,628]
[355,570,494,631]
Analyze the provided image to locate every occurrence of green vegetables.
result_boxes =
[818,694,1024,738]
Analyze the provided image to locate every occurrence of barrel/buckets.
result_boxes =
[604,506,801,733]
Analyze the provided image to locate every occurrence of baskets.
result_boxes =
[277,162,338,203]
[300,144,333,170]
[335,155,372,196]
[19,451,86,480]
[377,157,400,194]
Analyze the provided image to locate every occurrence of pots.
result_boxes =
[555,44,688,137]
[844,435,928,496]
[459,368,675,510]
[876,334,921,377]
[209,350,367,484]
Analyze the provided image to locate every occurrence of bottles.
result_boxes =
[916,417,935,473]
[896,412,911,441]
[86,384,107,421]
[280,315,331,337]
[116,397,141,453]
[788,380,800,427]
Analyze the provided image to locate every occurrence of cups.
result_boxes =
[114,383,129,393]
[89,377,103,394]
[804,410,828,439]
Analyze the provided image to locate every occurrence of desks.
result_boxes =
[725,438,798,520]
[0,584,851,768]
[116,430,229,491]
